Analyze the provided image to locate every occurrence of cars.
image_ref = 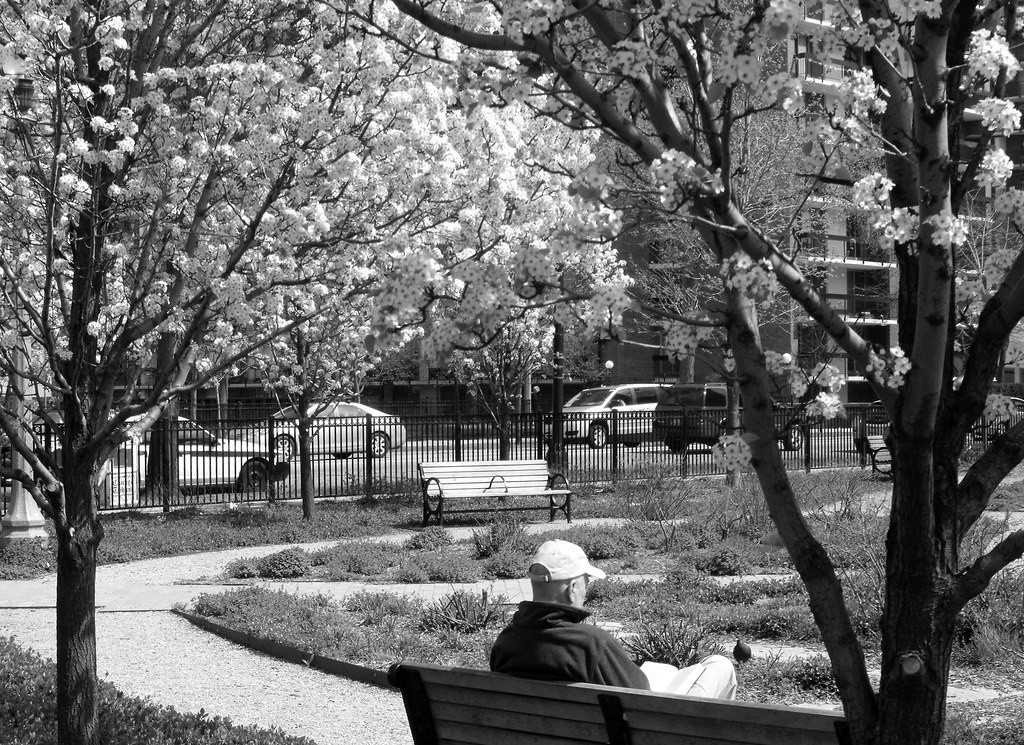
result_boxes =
[971,396,1024,439]
[223,400,407,459]
[851,399,893,436]
[34,412,291,493]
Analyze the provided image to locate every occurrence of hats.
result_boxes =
[525,539,606,583]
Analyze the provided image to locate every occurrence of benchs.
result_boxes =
[386,662,850,745]
[417,460,574,528]
[865,436,892,473]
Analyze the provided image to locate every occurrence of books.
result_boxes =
[639,661,706,695]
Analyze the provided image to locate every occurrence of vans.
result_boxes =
[541,383,675,449]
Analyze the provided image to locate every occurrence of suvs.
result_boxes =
[651,381,809,452]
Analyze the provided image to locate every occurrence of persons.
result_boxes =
[852,408,870,467]
[490,540,738,701]
